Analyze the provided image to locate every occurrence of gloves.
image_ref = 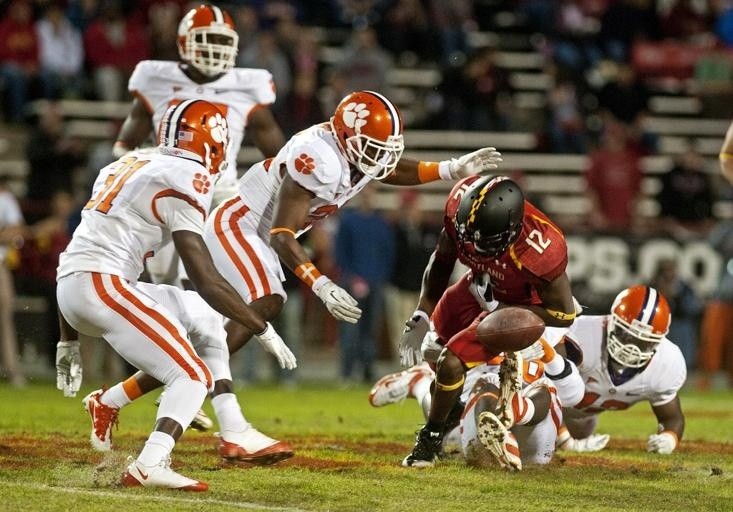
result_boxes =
[251,318,300,373]
[312,274,366,326]
[396,308,434,367]
[644,429,679,457]
[573,431,612,454]
[519,335,557,366]
[448,142,503,182]
[48,338,89,400]
[465,269,503,314]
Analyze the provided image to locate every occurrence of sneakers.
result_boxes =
[151,386,215,432]
[492,348,526,432]
[79,384,124,452]
[400,423,449,469]
[216,427,296,468]
[474,408,527,473]
[365,359,433,410]
[119,456,209,494]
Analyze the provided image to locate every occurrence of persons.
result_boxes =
[1,0,733,384]
[52,4,687,491]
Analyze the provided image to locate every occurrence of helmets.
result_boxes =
[452,170,526,260]
[610,282,672,348]
[156,94,230,177]
[173,3,237,65]
[330,86,406,164]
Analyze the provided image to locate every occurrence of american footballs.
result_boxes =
[475,307,545,352]
